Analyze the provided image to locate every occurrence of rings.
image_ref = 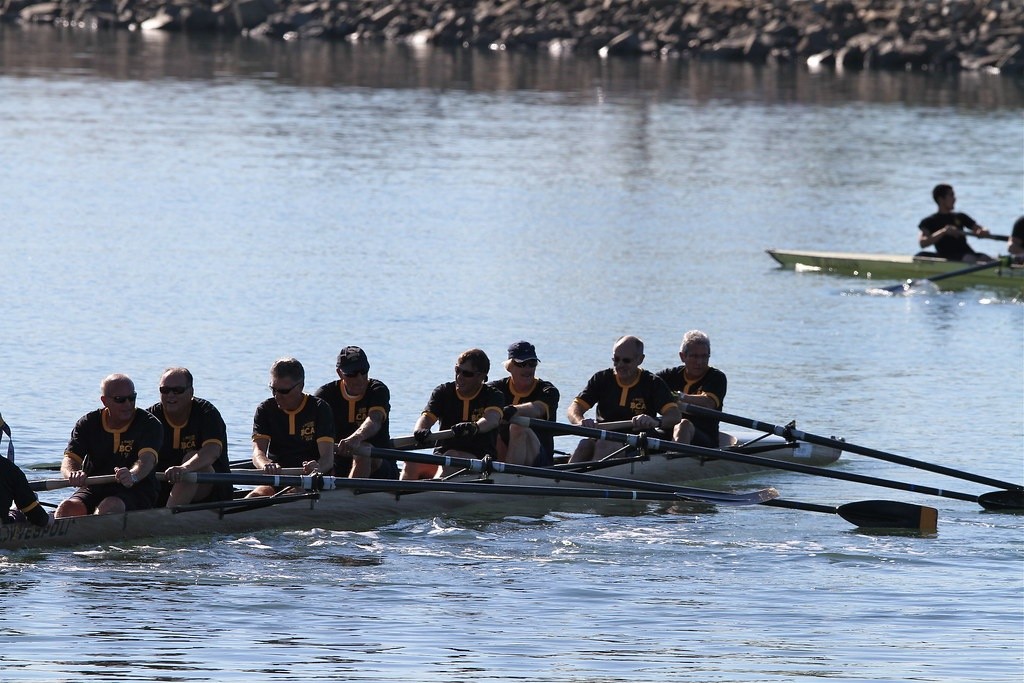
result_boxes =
[175,472,180,475]
[346,451,350,453]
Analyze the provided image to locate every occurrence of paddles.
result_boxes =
[33,427,455,470]
[27,475,119,492]
[507,413,1024,515]
[882,254,1014,291]
[355,442,939,539]
[675,399,1024,490]
[552,420,634,436]
[152,467,779,504]
[945,225,1009,241]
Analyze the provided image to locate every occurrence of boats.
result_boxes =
[0,433,851,561]
[765,248,1024,301]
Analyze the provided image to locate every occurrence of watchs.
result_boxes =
[131,474,138,482]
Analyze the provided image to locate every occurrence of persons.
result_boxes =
[0,412,50,523]
[135,366,234,510]
[54,374,165,519]
[399,349,504,480]
[482,339,560,468]
[915,184,993,262]
[565,334,682,463]
[657,330,728,450]
[1008,214,1024,264]
[242,357,334,498]
[311,346,400,478]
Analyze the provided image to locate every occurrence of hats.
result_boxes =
[335,346,370,374]
[509,340,541,363]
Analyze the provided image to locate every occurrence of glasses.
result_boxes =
[344,365,368,378]
[511,358,537,369]
[611,355,640,364]
[455,363,485,378]
[159,385,190,394]
[268,382,302,395]
[105,392,137,403]
[685,353,711,359]
